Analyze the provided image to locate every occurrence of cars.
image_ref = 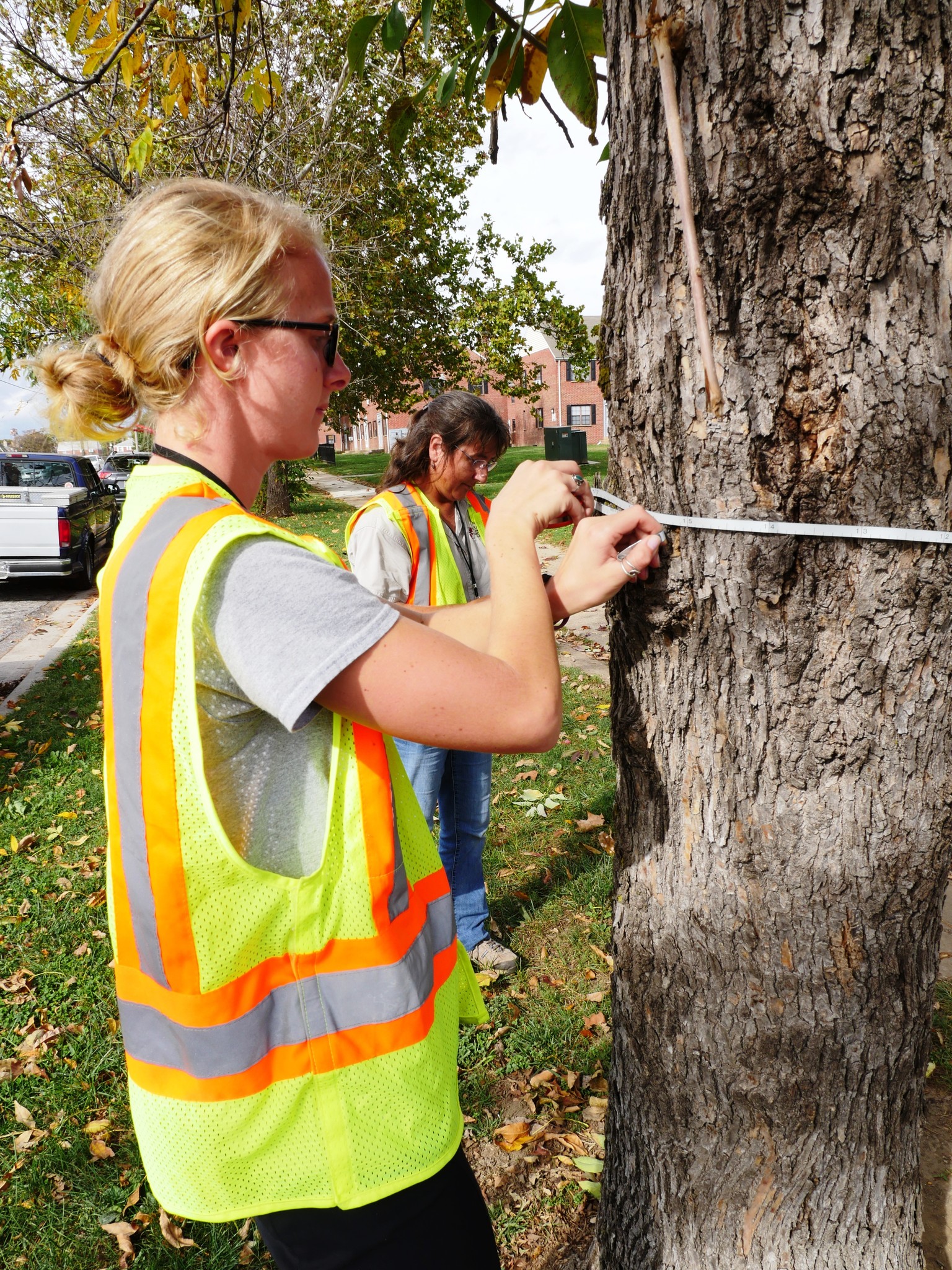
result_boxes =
[83,454,105,473]
[20,462,67,482]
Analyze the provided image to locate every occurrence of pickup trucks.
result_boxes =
[0,450,121,590]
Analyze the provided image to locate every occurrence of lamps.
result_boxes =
[551,408,554,413]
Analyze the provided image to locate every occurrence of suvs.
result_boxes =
[96,451,152,511]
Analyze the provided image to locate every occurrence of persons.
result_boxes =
[345,391,522,968]
[48,182,669,1270]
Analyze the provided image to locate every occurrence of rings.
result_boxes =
[620,556,641,578]
[570,474,585,493]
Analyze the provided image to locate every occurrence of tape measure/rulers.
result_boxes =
[590,487,951,545]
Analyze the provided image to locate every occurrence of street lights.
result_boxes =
[10,428,18,452]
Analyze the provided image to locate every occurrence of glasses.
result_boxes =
[453,444,498,474]
[180,319,340,372]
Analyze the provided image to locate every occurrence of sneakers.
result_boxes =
[467,938,521,975]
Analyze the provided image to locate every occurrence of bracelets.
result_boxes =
[541,574,569,631]
